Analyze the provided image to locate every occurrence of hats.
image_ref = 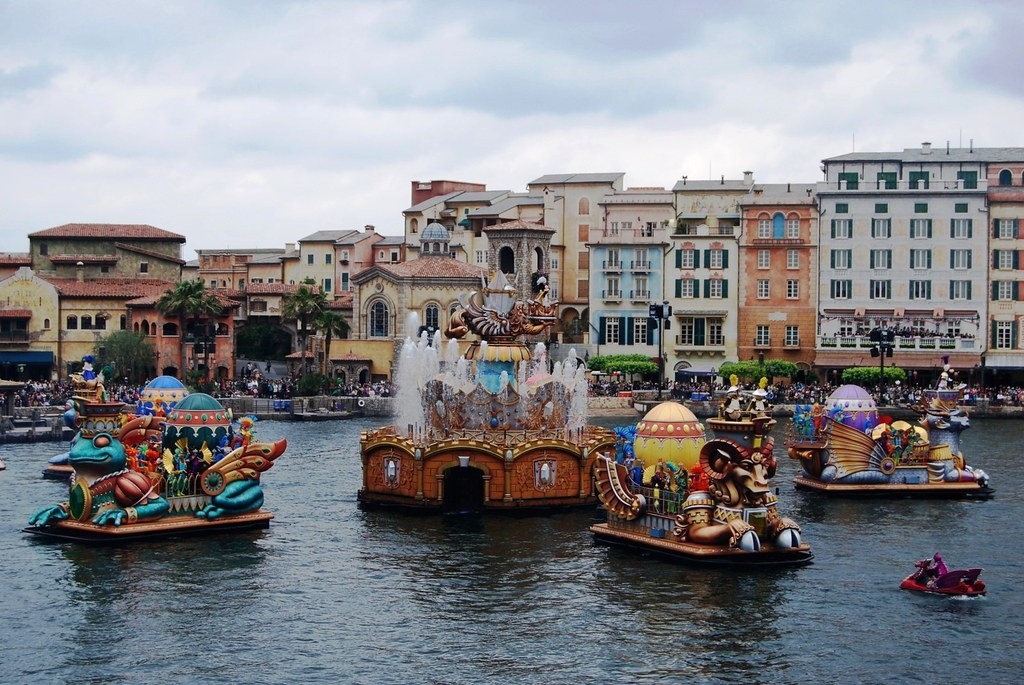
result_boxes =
[933,552,942,561]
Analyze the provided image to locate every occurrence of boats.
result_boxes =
[588,401,815,567]
[294,408,359,421]
[21,355,288,547]
[632,398,751,417]
[900,558,988,597]
[706,409,778,434]
[786,383,997,500]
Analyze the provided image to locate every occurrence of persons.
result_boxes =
[0,359,1024,408]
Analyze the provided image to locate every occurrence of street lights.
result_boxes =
[194,325,216,394]
[647,304,672,400]
[869,330,894,407]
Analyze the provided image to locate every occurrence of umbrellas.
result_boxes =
[285,351,315,358]
